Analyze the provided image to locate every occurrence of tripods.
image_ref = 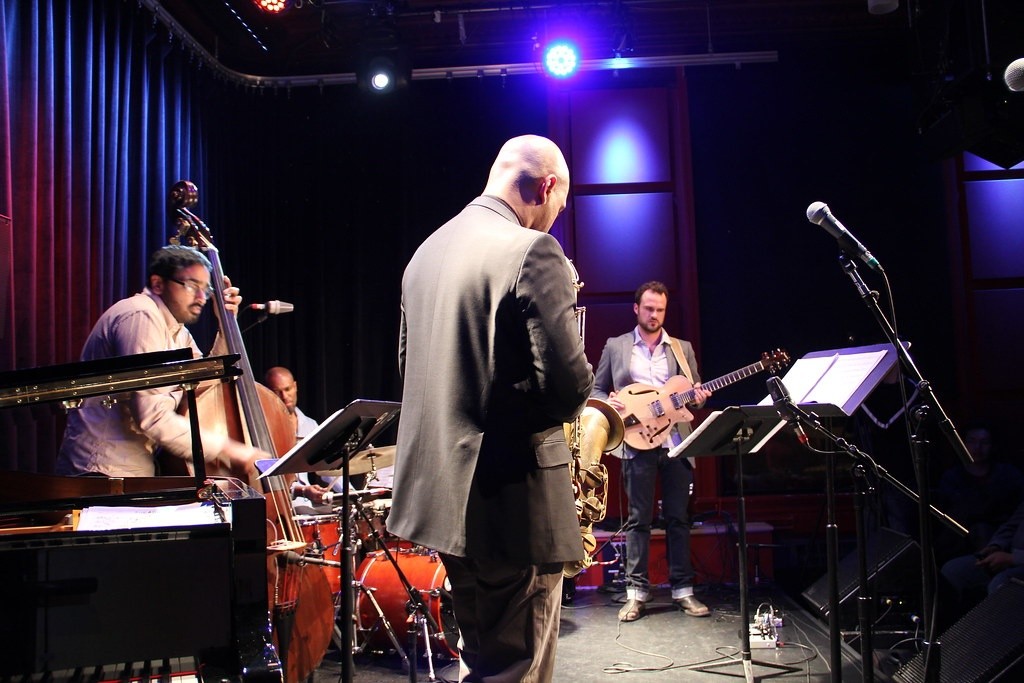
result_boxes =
[333,458,412,677]
[670,406,803,683]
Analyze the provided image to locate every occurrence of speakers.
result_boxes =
[800,528,1024,683]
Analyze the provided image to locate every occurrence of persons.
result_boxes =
[587,279,712,621]
[56,245,272,478]
[384,135,594,683]
[266,367,356,509]
[870,361,1024,669]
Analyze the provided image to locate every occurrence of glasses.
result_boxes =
[157,274,214,299]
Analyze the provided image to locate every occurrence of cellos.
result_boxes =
[169,180,334,683]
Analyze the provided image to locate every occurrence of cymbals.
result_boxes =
[315,445,397,477]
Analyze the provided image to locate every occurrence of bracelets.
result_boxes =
[301,486,307,498]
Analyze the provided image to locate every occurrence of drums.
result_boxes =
[333,486,399,544]
[353,549,459,658]
[291,513,343,594]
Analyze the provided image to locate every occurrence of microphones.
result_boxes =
[279,550,341,567]
[1004,58,1024,91]
[251,300,294,314]
[807,201,885,275]
[766,377,810,449]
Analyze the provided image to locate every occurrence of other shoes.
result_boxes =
[617,599,646,621]
[672,595,710,616]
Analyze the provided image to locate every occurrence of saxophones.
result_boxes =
[563,259,625,578]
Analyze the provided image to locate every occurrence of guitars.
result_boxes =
[612,348,791,450]
